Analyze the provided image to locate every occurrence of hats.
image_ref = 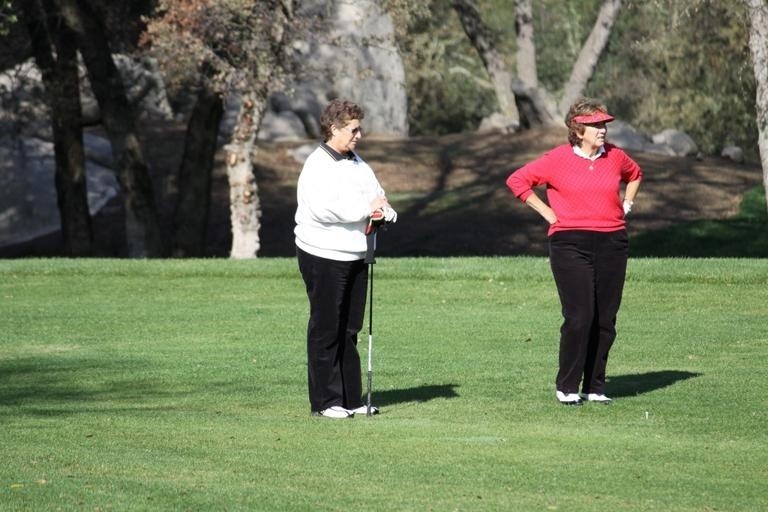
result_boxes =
[573,109,615,124]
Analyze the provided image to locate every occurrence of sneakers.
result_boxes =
[313,406,355,418]
[580,390,611,402]
[348,404,378,415]
[556,390,583,406]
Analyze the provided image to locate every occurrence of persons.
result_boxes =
[291,97,399,420]
[503,98,644,406]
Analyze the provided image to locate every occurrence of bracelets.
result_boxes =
[622,197,633,205]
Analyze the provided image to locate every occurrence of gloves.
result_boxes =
[383,205,398,224]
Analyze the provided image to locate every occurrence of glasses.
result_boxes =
[346,127,358,133]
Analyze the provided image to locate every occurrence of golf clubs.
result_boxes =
[367,219,398,422]
